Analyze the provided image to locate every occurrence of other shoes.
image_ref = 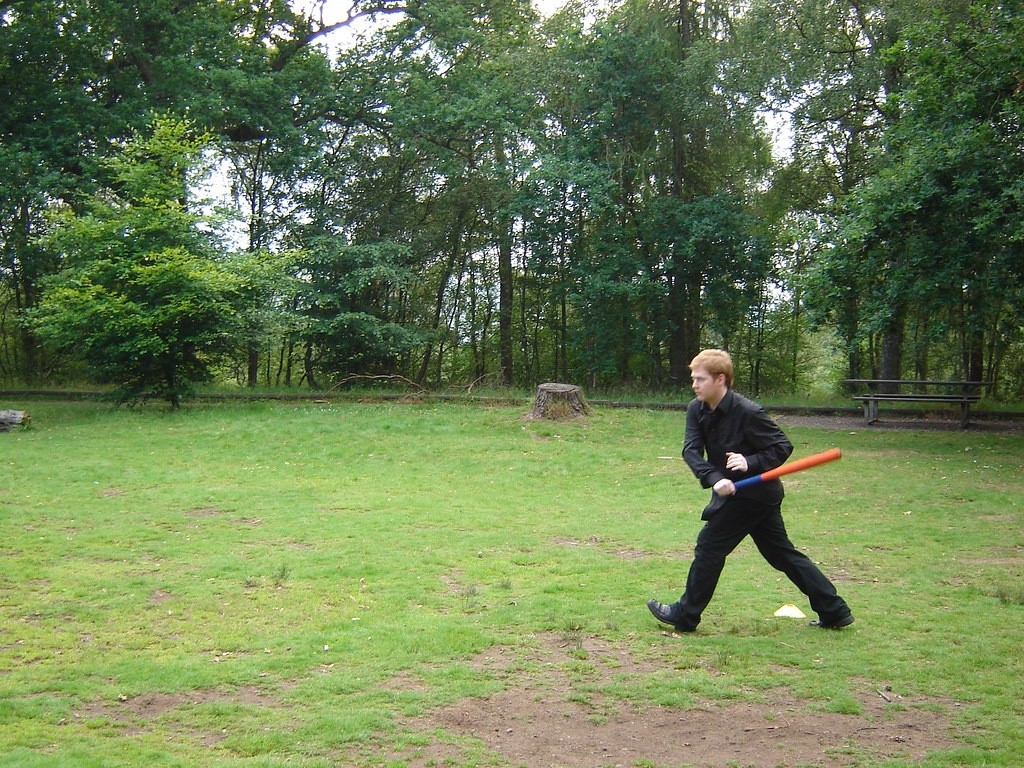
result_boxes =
[647,598,694,633]
[808,610,855,631]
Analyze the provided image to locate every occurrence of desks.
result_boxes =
[843,378,982,424]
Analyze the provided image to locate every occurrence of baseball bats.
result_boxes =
[734,447,842,488]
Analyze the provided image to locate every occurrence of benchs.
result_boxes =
[862,393,981,418]
[852,396,978,429]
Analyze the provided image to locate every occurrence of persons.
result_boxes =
[647,349,856,631]
